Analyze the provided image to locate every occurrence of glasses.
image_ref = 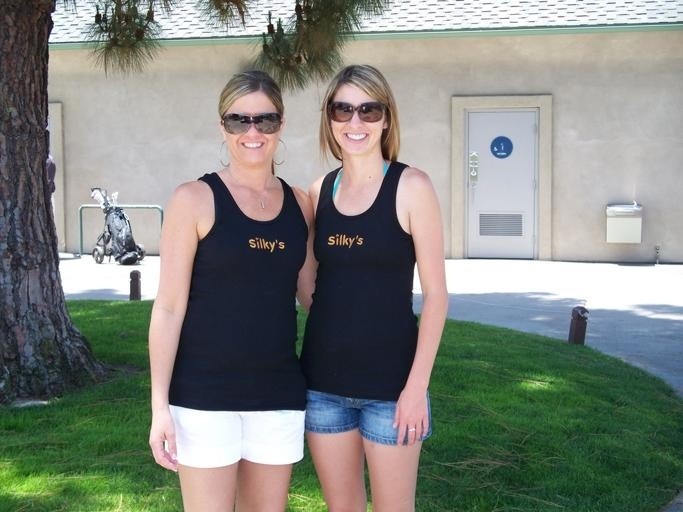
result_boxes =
[327,102,382,123]
[221,113,280,135]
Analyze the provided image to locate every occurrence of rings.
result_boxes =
[408,428,416,432]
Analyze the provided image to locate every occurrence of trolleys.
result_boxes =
[89,186,144,265]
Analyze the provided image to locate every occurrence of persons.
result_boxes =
[148,68,314,512]
[301,63,448,512]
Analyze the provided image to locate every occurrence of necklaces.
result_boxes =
[225,164,279,211]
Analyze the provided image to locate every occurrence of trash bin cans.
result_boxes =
[606,205,643,244]
